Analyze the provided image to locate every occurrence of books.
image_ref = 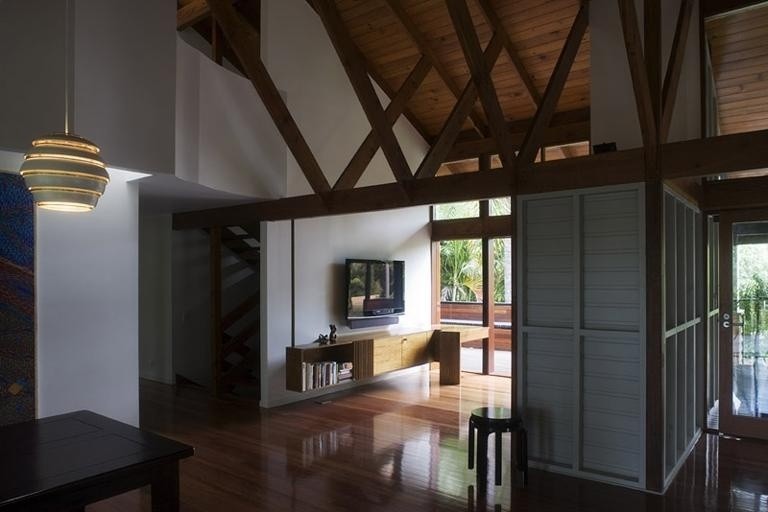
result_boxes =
[300,358,356,391]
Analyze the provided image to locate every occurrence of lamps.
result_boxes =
[18,2,110,215]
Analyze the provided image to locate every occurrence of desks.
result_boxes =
[1,410,196,512]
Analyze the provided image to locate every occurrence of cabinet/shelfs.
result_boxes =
[285,324,490,393]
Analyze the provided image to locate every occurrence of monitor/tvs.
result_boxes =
[344,258,405,319]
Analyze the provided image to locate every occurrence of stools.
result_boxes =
[467,407,529,489]
